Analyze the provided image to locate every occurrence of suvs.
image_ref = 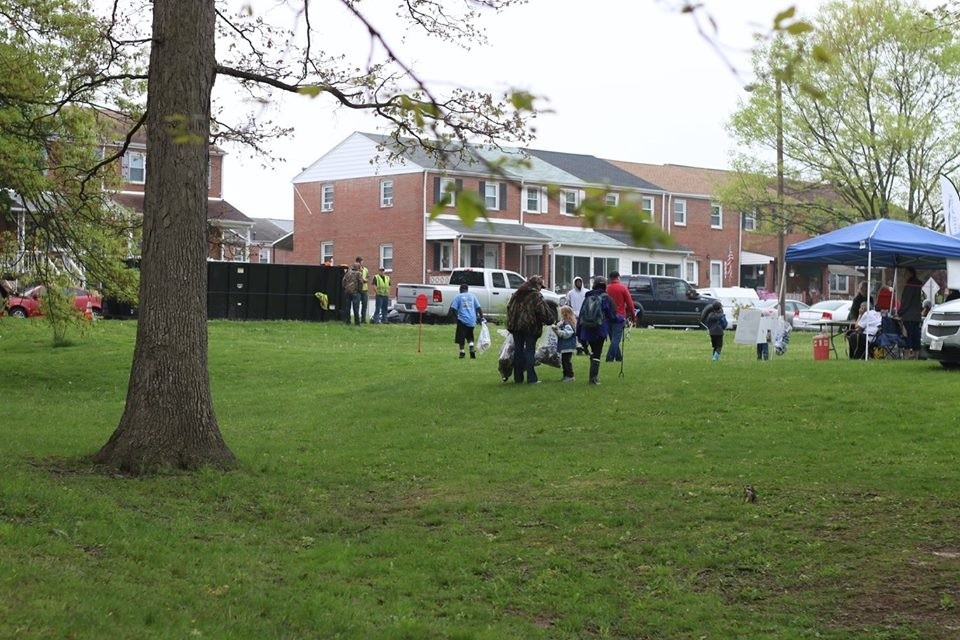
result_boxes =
[919,297,960,368]
[620,274,722,329]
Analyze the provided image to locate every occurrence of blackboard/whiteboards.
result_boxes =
[733,308,761,345]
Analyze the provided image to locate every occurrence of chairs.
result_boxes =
[859,316,899,360]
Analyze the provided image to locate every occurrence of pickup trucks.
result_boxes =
[394,267,567,325]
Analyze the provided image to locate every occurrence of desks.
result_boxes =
[808,319,856,361]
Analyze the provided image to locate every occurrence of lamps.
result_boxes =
[759,264,764,275]
[789,262,794,277]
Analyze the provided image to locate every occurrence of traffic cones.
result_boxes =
[84,297,93,320]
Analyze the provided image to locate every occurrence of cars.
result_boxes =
[6,282,103,319]
[750,298,810,325]
[791,300,854,334]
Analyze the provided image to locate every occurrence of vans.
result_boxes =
[696,286,761,331]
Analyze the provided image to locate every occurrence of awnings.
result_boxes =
[828,265,864,276]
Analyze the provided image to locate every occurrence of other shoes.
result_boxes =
[530,380,543,386]
[561,376,577,383]
[712,352,720,360]
[470,350,476,359]
[458,352,467,359]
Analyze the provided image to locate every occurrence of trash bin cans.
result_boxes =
[813,335,829,360]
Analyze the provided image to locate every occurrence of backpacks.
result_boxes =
[580,289,610,330]
[343,270,360,297]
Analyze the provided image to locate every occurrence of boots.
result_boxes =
[589,358,606,384]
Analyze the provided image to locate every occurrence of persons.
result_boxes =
[506,276,555,383]
[606,272,637,362]
[342,263,363,326]
[921,300,933,316]
[321,256,331,266]
[356,256,370,323]
[706,302,728,360]
[450,284,484,359]
[851,282,874,320]
[850,301,882,360]
[898,267,923,359]
[565,277,592,356]
[576,275,625,384]
[944,287,960,302]
[552,306,578,382]
[372,267,392,324]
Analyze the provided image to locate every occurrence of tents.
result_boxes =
[771,219,960,361]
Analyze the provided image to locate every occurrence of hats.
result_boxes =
[711,300,722,311]
[529,274,547,289]
[351,262,362,270]
[379,267,385,270]
[593,275,608,285]
